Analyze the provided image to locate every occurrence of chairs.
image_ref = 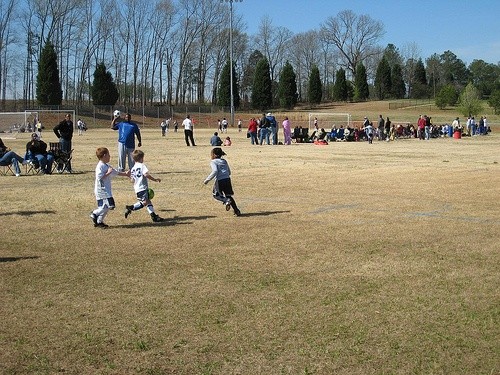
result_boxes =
[0,142,74,176]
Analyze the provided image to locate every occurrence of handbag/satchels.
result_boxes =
[247,131,251,138]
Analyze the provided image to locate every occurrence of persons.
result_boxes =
[238,119,242,132]
[77,118,87,136]
[293,114,464,145]
[125,150,164,223]
[161,121,167,136]
[282,117,292,145]
[209,132,223,146]
[166,118,171,131]
[89,147,130,229]
[53,113,74,174]
[0,138,30,176]
[182,114,197,146]
[216,118,229,134]
[111,114,142,172]
[27,116,41,133]
[173,120,178,133]
[247,113,278,145]
[466,116,487,136]
[224,137,231,146]
[26,134,53,175]
[204,148,241,217]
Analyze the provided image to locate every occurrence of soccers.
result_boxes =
[113,110,120,117]
[149,188,154,200]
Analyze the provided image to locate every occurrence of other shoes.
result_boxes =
[89,211,99,227]
[124,205,134,219]
[22,160,31,166]
[225,198,232,211]
[152,214,165,223]
[93,221,109,228]
[15,173,20,177]
[232,210,241,217]
